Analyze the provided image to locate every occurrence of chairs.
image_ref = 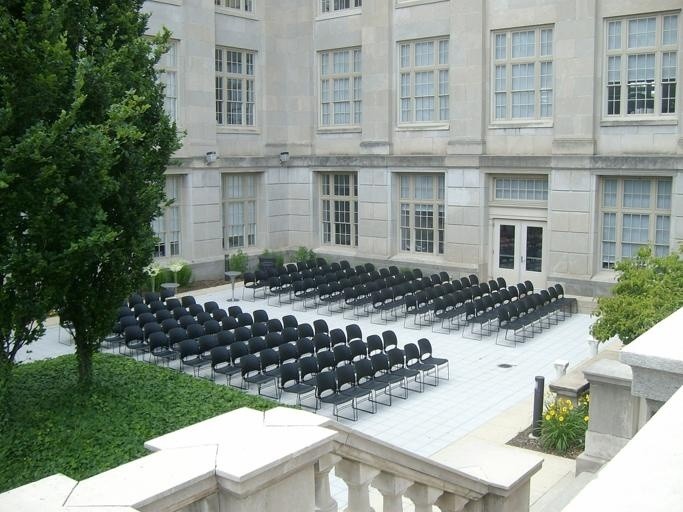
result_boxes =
[239,258,574,348]
[58,292,450,420]
